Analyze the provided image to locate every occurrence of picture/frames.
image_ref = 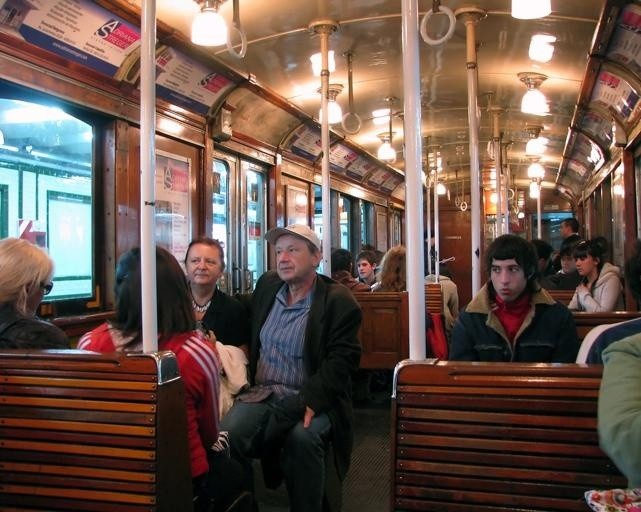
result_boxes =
[376,211,388,254]
[285,184,309,228]
[155,148,193,277]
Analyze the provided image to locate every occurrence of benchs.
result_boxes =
[1,286,640,511]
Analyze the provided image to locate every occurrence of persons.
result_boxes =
[0,236,72,349]
[183,237,248,421]
[208,223,364,511]
[77,244,254,505]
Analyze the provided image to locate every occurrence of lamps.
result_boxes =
[315,83,345,125]
[190,0,231,48]
[376,132,448,196]
[509,0,556,201]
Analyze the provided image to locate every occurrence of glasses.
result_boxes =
[31,278,54,295]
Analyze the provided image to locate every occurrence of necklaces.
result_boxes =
[189,286,211,313]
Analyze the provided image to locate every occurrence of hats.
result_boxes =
[264,224,323,251]
